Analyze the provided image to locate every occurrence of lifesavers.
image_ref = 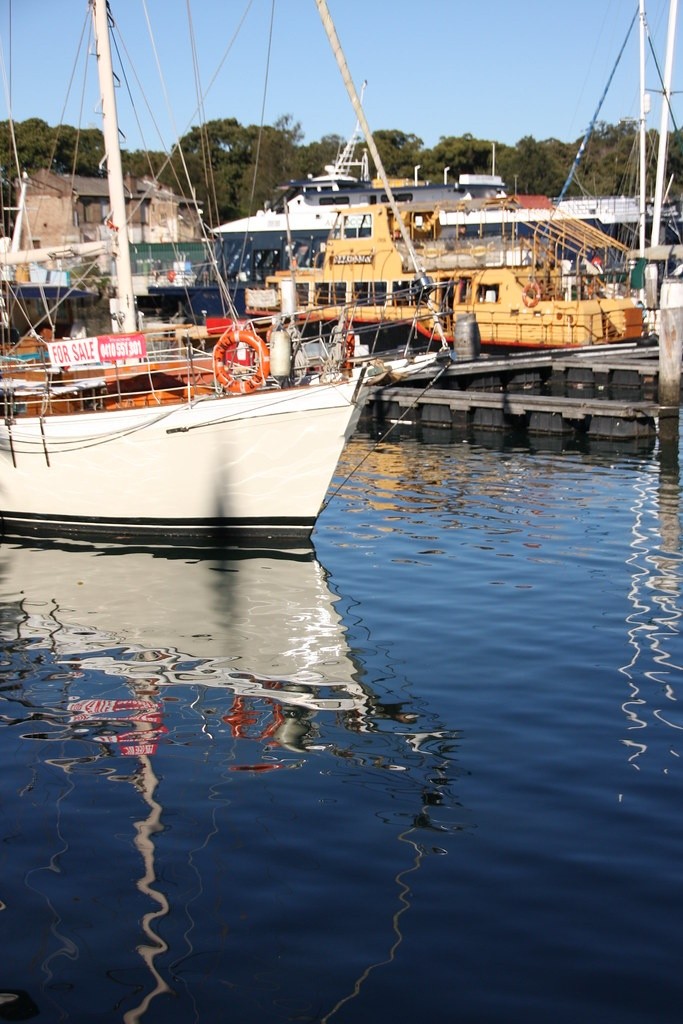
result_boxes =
[212,329,271,394]
[521,284,542,308]
[341,322,356,368]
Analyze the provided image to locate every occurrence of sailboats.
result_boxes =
[0,535,372,1024]
[1,0,682,552]
